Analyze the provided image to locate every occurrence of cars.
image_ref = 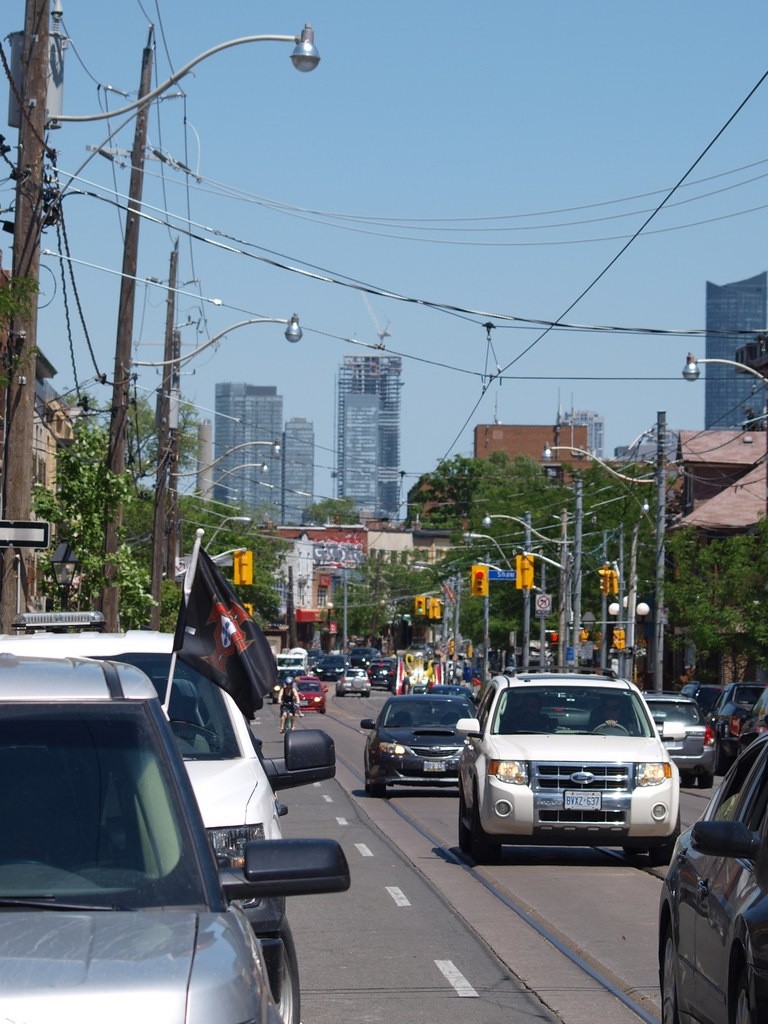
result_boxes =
[349,647,397,693]
[737,686,768,759]
[269,647,328,714]
[427,685,474,704]
[404,643,435,664]
[359,694,478,798]
[335,669,371,698]
[678,681,732,720]
[640,689,717,789]
[314,654,348,682]
[1,628,339,930]
[657,730,767,1024]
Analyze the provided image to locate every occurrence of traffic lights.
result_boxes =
[416,598,424,613]
[475,571,485,594]
[548,632,560,652]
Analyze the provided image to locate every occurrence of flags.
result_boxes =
[172,546,279,722]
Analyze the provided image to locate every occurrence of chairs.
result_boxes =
[440,712,460,725]
[391,711,412,727]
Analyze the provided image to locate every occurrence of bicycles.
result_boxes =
[277,701,301,735]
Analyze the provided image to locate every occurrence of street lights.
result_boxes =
[540,410,667,694]
[1,21,323,630]
[149,439,284,628]
[460,509,569,672]
[609,595,651,678]
[97,313,305,628]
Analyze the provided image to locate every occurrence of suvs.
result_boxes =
[0,652,351,1023]
[455,665,683,866]
[704,681,764,776]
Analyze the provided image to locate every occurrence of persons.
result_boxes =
[593,694,636,735]
[401,669,411,695]
[503,694,549,732]
[278,677,304,732]
[672,673,689,694]
